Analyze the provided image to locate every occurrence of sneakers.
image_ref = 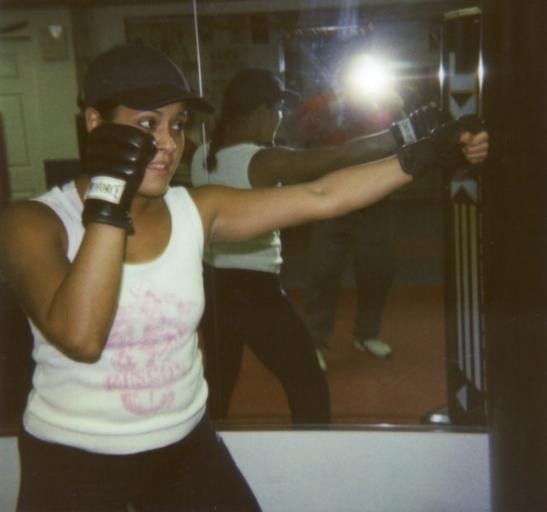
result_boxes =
[354,338,393,359]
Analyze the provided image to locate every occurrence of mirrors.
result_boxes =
[1,4,488,432]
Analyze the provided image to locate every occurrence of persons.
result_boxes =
[290,81,399,372]
[0,42,496,512]
[190,67,444,422]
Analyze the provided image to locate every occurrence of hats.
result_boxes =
[79,43,216,119]
[224,66,302,116]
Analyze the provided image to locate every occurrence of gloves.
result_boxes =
[80,122,158,237]
[396,113,490,178]
[389,103,448,146]
